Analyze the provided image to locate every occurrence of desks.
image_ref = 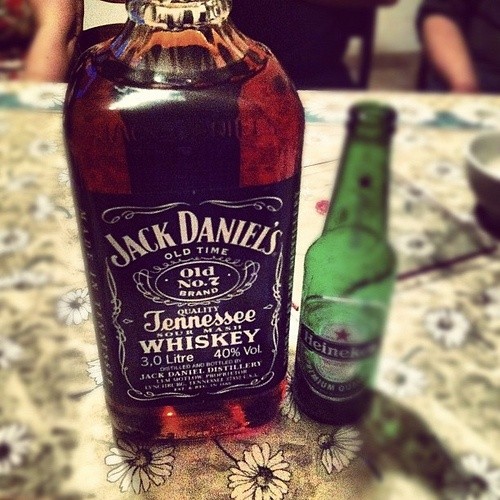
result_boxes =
[0,80,500,500]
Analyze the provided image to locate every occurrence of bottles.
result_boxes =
[63,0,306,444]
[291,102,397,426]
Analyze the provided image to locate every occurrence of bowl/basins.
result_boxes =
[465,130,500,216]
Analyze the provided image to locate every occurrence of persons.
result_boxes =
[0,0,84,83]
[415,0,500,96]
